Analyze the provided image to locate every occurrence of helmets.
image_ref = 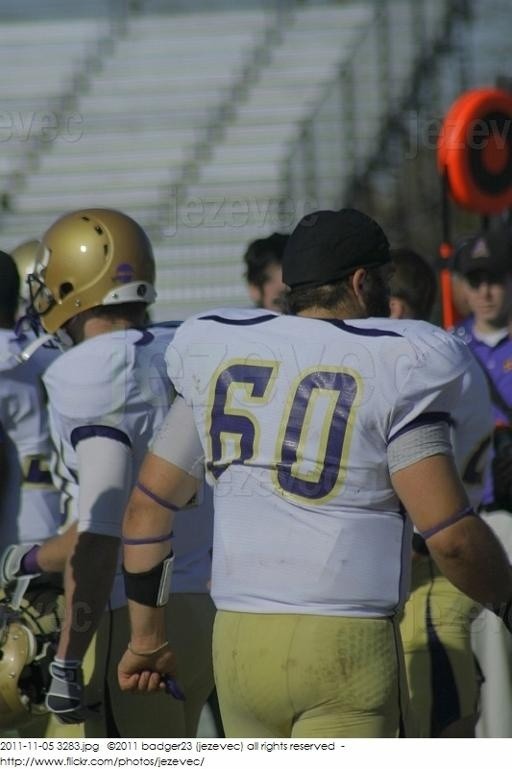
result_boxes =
[10,208,155,335]
[0,622,48,730]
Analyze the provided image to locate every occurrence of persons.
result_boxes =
[0,207,512,738]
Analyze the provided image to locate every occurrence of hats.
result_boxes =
[453,232,511,273]
[282,208,390,291]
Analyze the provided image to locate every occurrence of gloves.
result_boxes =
[46,657,89,726]
[0,542,43,584]
